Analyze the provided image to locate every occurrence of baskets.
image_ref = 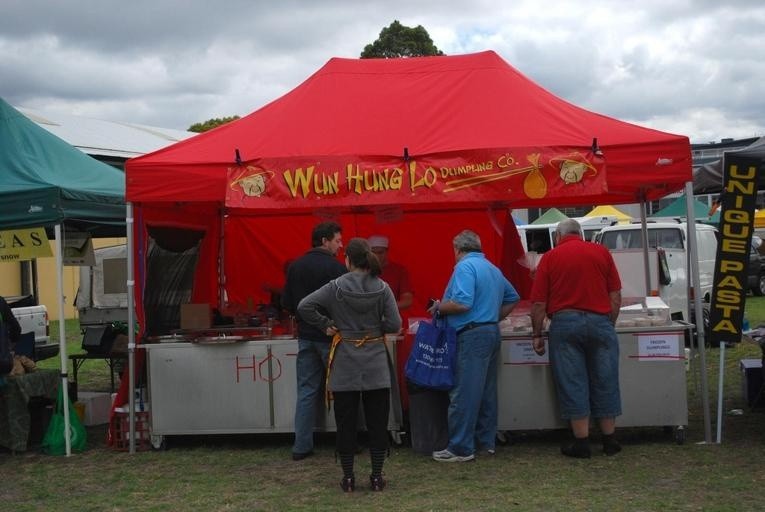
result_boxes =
[111,409,150,451]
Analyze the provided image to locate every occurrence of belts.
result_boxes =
[457,321,498,331]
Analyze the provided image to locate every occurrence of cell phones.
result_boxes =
[426,299,437,308]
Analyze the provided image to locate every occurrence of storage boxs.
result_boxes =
[739,358,763,401]
[78,391,112,427]
[180,304,213,329]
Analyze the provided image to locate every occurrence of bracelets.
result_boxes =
[533,335,542,339]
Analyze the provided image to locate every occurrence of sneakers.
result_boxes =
[602,437,624,455]
[291,448,316,461]
[559,436,593,459]
[431,446,477,461]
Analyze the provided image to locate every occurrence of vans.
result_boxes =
[517,212,765,342]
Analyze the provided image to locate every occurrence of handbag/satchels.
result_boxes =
[403,318,459,390]
[40,409,87,455]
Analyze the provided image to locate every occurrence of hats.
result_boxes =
[367,233,390,248]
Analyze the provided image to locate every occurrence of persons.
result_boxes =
[0,295,21,374]
[426,230,521,462]
[297,238,402,492]
[530,220,623,458]
[279,223,364,461]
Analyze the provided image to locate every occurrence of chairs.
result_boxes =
[15,330,36,365]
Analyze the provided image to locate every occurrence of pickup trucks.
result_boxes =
[7,302,61,365]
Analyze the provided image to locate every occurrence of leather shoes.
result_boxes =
[341,477,358,491]
[370,474,385,490]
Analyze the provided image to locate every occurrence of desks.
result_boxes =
[69,354,126,391]
